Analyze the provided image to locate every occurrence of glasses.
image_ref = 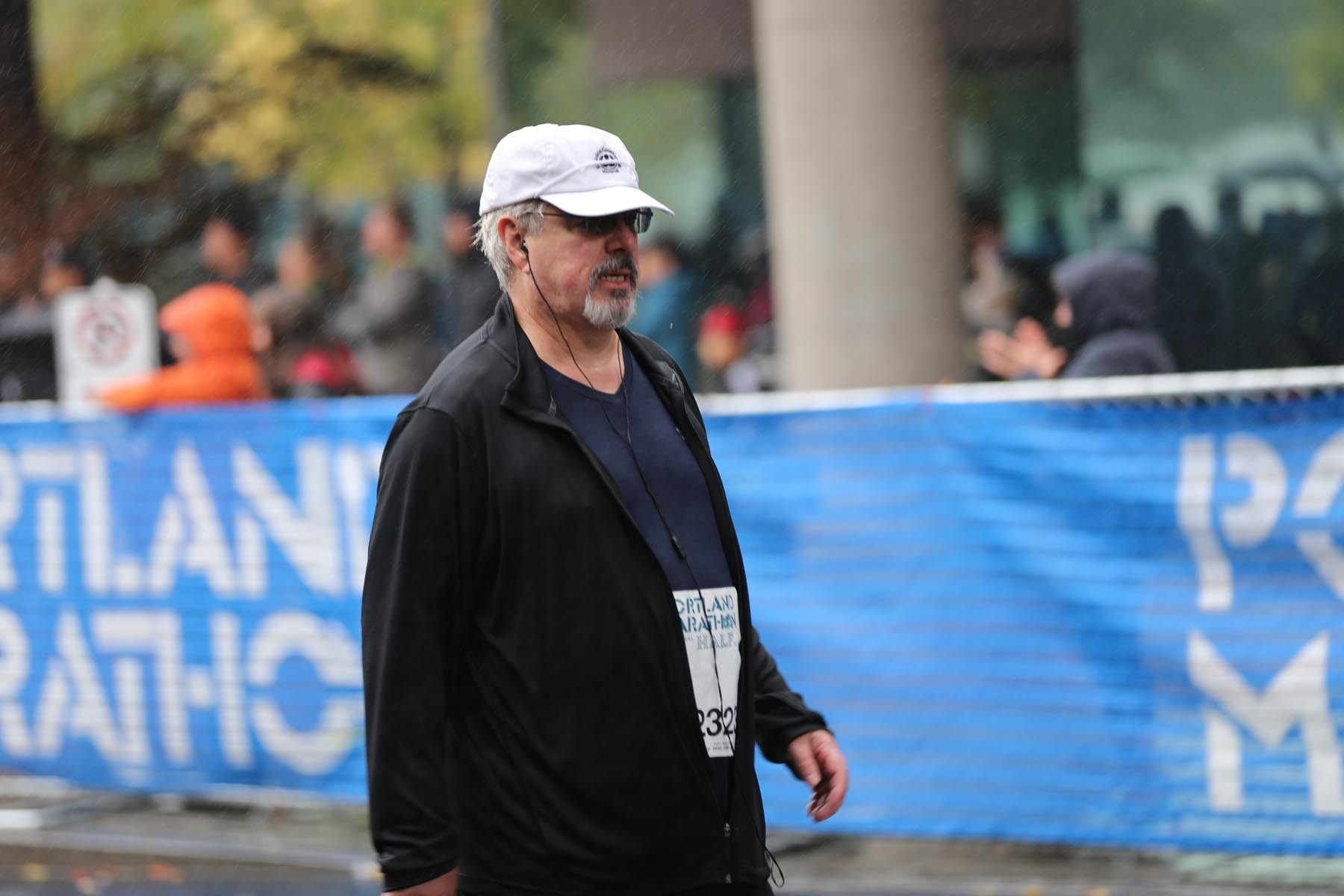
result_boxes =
[533,207,654,234]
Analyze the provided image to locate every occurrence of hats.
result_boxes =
[479,123,676,219]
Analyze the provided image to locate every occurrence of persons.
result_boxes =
[959,177,1344,383]
[626,217,780,397]
[0,181,503,413]
[361,123,849,896]
